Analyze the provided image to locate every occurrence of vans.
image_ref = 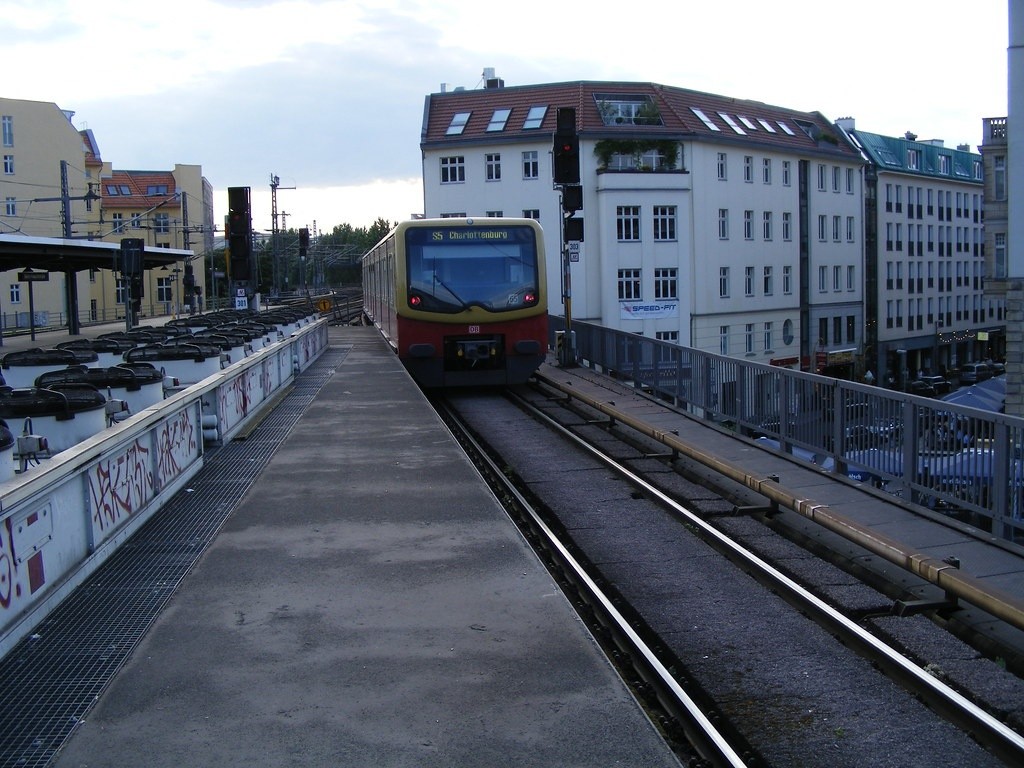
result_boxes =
[958,363,1005,386]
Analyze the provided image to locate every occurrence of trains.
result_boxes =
[361,217,550,388]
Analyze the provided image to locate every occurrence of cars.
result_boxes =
[824,398,867,422]
[831,407,934,452]
[890,375,952,398]
[741,415,797,439]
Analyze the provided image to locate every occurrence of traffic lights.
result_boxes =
[299,248,306,256]
[194,285,202,296]
[225,185,251,258]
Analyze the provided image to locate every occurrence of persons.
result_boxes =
[926,415,975,451]
[985,351,1003,378]
[917,369,924,381]
[889,376,895,387]
[437,258,477,286]
